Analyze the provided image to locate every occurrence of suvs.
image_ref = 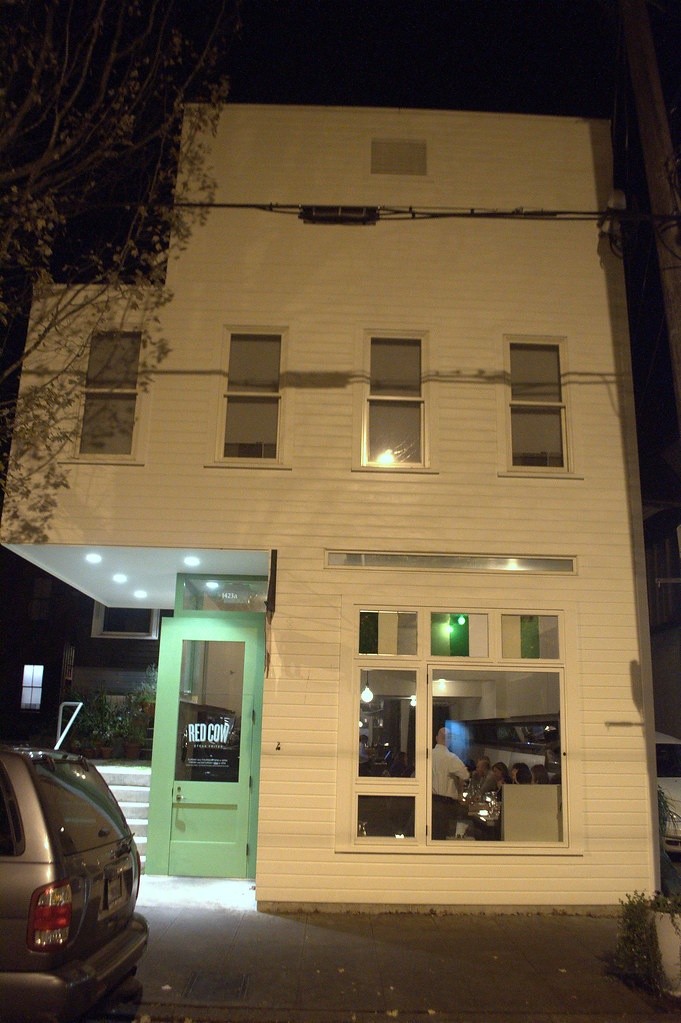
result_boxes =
[0,743,152,1023]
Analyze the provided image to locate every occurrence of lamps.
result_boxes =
[410,698,416,706]
[361,671,374,702]
[458,615,466,625]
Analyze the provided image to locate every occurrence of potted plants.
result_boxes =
[61,664,159,763]
[613,891,681,1000]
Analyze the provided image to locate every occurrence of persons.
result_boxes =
[431,726,470,840]
[464,755,549,799]
[390,751,407,777]
[358,735,378,777]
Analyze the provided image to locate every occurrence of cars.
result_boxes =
[655,731,681,854]
